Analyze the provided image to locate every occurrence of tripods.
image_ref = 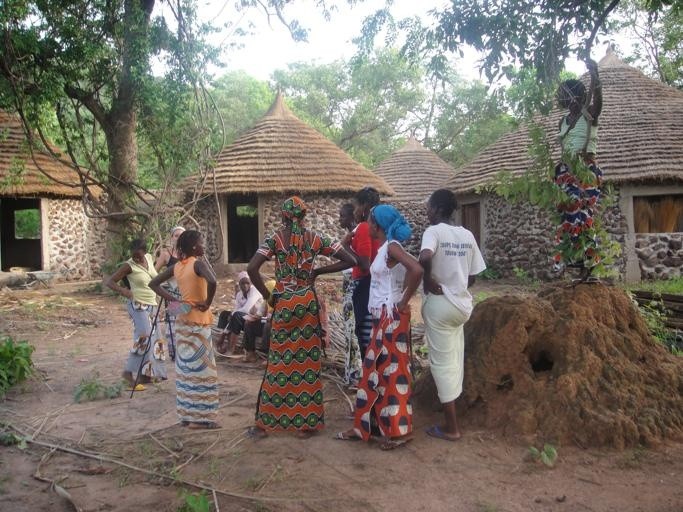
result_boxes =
[130,284,182,399]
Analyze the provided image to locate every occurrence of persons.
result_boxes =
[415,188,488,439]
[239,279,277,363]
[146,229,220,430]
[245,193,358,440]
[215,271,264,354]
[333,199,356,394]
[153,226,185,363]
[552,58,610,285]
[105,237,168,391]
[342,185,385,358]
[332,204,425,450]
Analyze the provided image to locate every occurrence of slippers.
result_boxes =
[248,426,268,439]
[379,433,414,450]
[126,383,147,391]
[240,355,257,363]
[291,429,312,439]
[181,419,223,430]
[426,424,461,441]
[334,427,363,441]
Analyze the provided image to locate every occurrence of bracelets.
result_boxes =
[265,293,273,303]
[591,85,600,91]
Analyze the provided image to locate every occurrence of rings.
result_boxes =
[438,287,440,290]
